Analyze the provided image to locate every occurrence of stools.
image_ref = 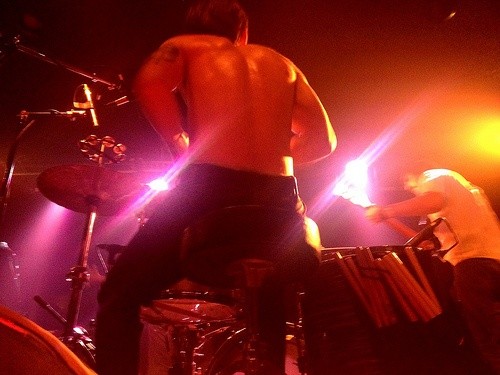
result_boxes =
[178,205,322,374]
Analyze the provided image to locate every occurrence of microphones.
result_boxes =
[0,242,14,254]
[403,218,442,247]
[104,95,129,109]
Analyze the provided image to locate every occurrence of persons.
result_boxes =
[93,0,336,375]
[365,159,499,374]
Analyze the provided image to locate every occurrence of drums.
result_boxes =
[139,298,237,375]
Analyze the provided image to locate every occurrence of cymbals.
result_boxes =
[37,164,145,215]
